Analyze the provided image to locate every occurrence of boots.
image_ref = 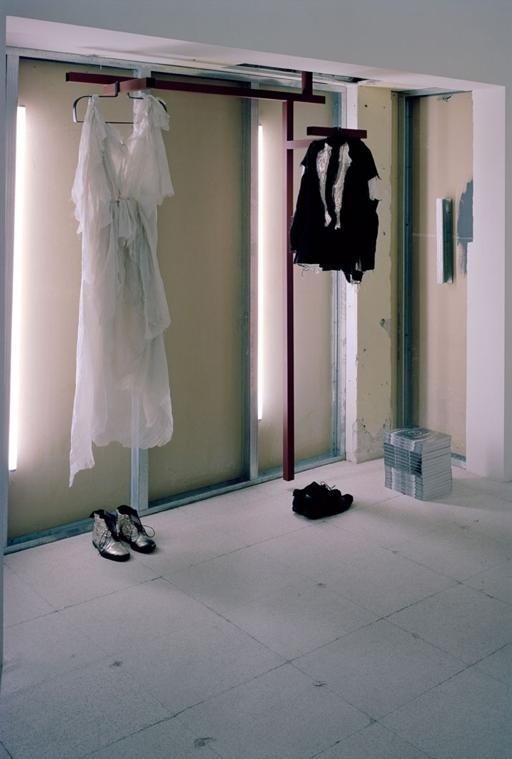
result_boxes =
[116,505,156,554]
[90,508,130,562]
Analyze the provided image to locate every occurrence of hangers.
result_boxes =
[72,81,168,124]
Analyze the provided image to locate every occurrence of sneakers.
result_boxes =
[302,488,354,520]
[292,481,341,513]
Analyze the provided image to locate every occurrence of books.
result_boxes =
[383,427,454,499]
